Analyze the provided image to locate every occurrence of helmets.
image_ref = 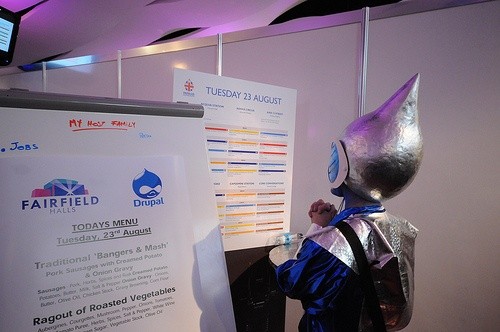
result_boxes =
[328,73,424,201]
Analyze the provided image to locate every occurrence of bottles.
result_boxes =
[275,233,303,245]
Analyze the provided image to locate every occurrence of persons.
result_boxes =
[275,71,424,332]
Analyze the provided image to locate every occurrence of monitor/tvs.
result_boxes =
[0,6,21,66]
